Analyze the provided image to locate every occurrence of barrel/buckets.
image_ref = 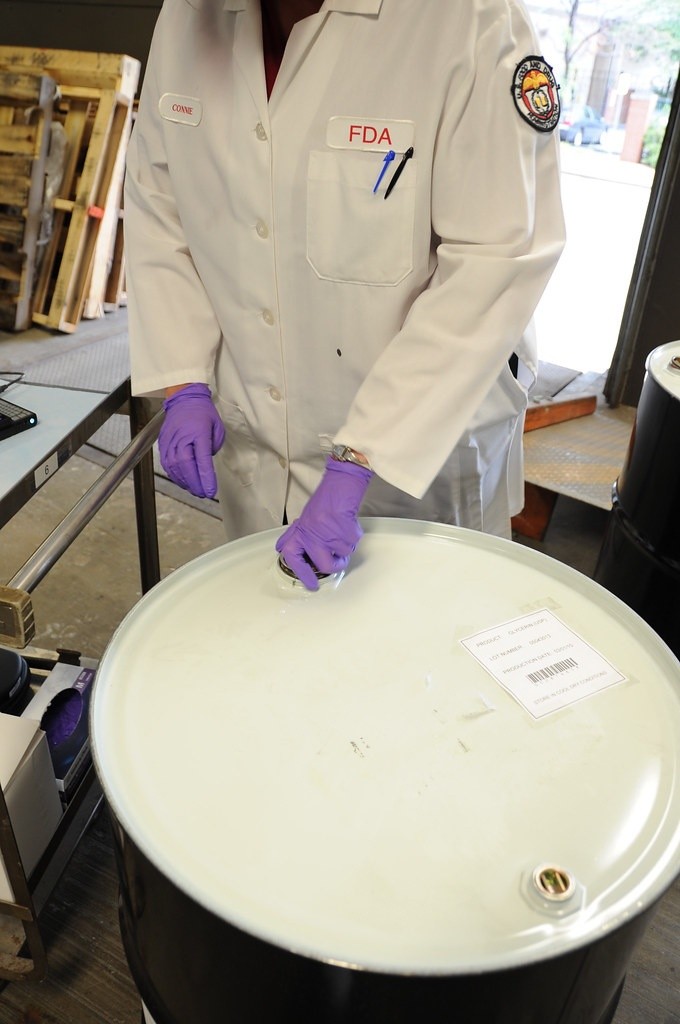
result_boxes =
[594,340,680,662]
[89,515,680,1024]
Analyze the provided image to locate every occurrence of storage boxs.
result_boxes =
[0,712,64,903]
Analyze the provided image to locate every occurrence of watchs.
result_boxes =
[332,444,374,472]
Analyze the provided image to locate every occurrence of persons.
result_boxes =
[124,0,568,594]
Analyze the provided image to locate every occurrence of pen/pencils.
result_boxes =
[372,146,414,199]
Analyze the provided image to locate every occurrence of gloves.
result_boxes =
[275,455,375,591]
[158,383,225,498]
